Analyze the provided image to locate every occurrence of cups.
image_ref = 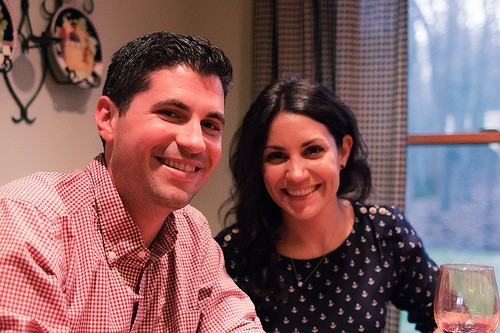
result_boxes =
[434,264,500,333]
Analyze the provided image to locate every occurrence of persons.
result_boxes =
[0,31,266,333]
[214,74,472,333]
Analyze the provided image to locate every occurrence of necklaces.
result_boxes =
[285,214,342,287]
